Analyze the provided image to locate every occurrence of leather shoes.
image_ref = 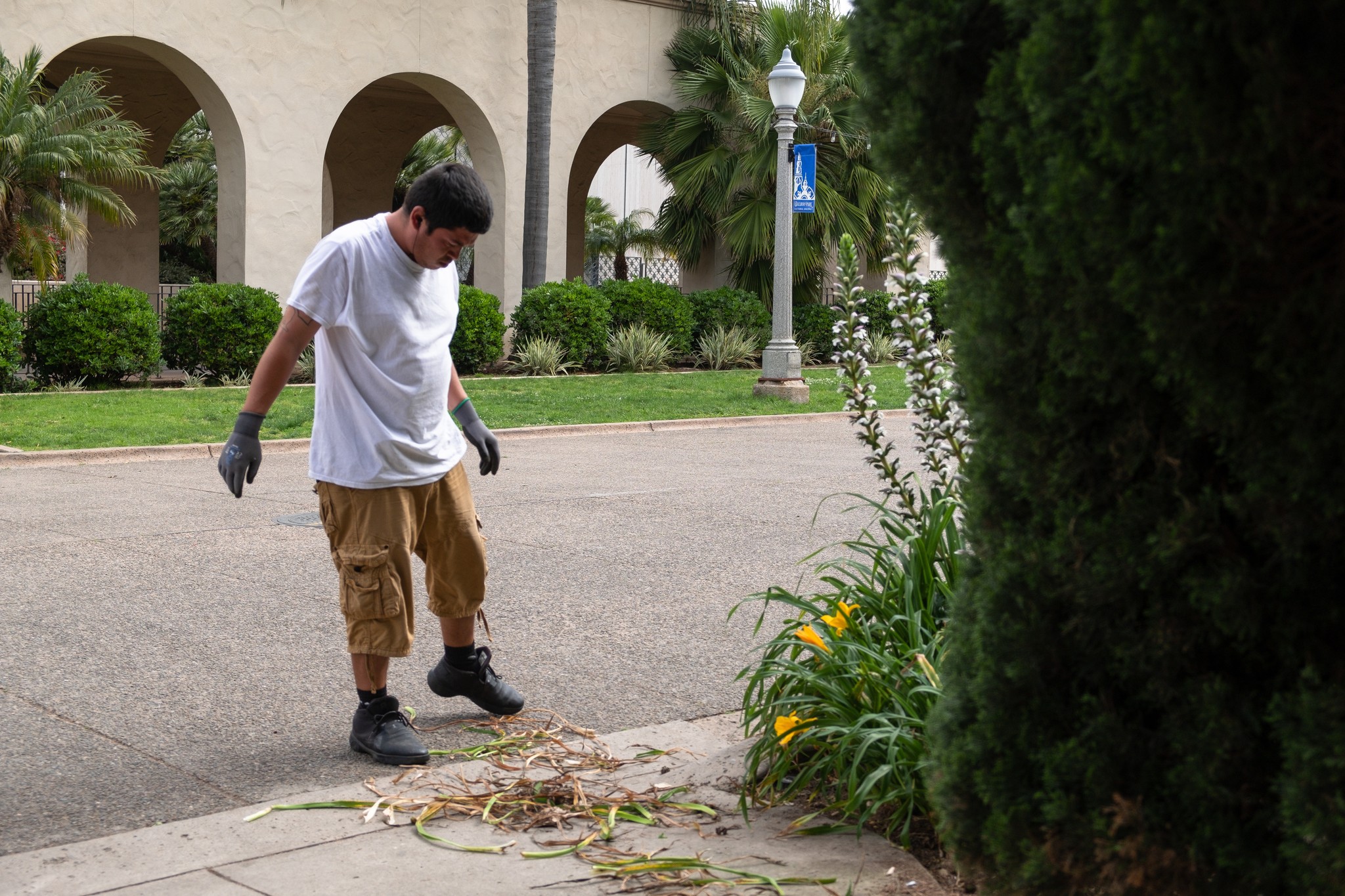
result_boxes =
[349,695,430,765]
[427,645,525,715]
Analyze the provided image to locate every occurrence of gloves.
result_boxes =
[452,398,501,476]
[218,411,266,499]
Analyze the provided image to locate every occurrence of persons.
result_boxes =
[218,163,525,766]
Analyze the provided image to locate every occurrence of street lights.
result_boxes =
[752,44,810,402]
